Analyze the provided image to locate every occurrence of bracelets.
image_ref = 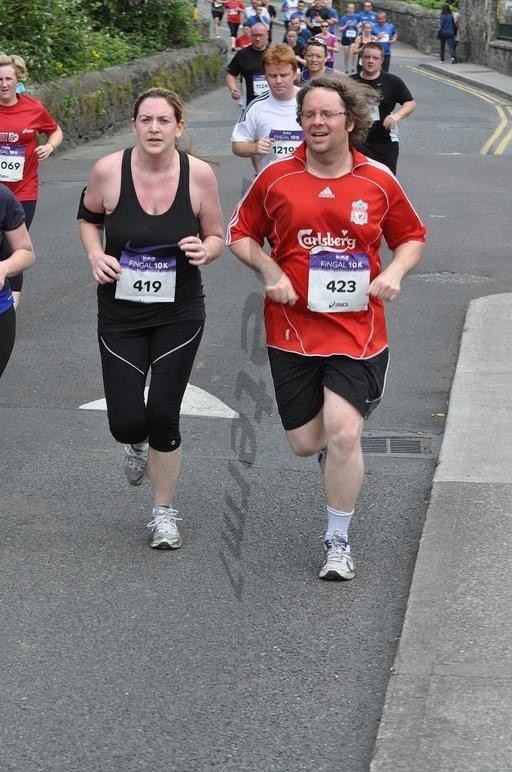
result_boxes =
[48,140,56,152]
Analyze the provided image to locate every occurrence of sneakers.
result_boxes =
[123,444,149,487]
[318,447,327,473]
[439,60,445,64]
[344,69,355,74]
[451,57,455,64]
[149,506,182,549]
[318,529,356,581]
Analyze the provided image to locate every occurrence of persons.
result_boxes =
[76,87,225,551]
[223,72,426,582]
[2,179,38,381]
[204,0,418,181]
[436,3,459,65]
[1,52,66,314]
[10,54,29,97]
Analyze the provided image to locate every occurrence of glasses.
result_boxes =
[297,109,349,119]
